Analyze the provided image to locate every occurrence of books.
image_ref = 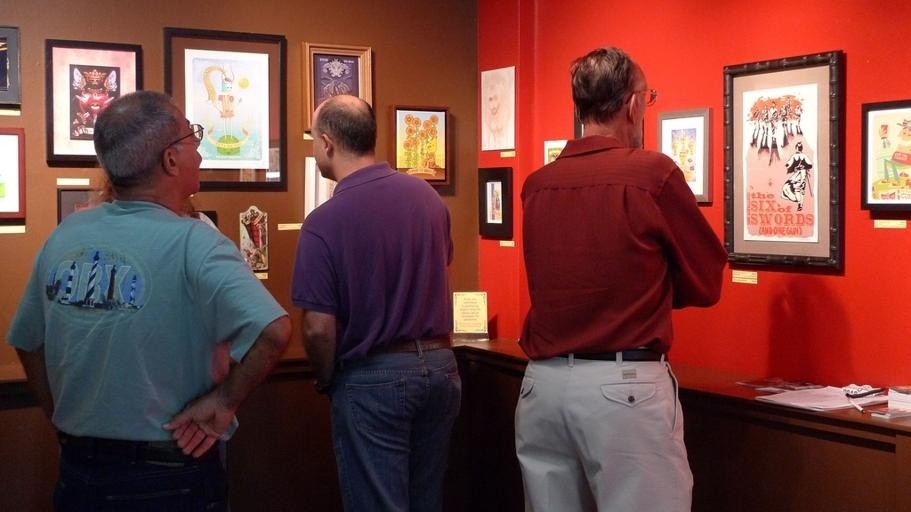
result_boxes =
[753,385,911,421]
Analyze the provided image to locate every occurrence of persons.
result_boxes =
[6,91,295,512]
[511,45,727,511]
[289,92,462,511]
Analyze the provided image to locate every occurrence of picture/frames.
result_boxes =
[658,107,712,203]
[721,47,845,274]
[476,165,513,240]
[861,100,911,213]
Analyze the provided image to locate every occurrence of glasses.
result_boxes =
[167,124,203,148]
[626,89,656,105]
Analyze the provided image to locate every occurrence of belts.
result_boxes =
[95,442,184,463]
[560,349,668,362]
[390,337,450,353]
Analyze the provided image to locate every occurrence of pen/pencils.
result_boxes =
[847,396,867,414]
